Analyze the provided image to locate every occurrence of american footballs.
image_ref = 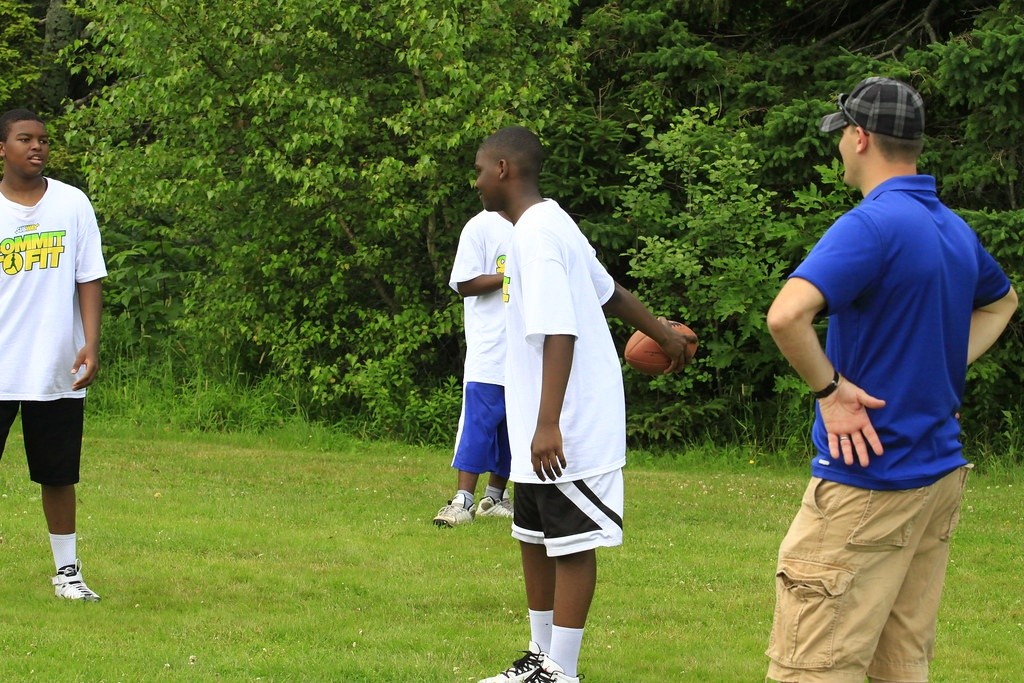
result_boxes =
[624,321,698,375]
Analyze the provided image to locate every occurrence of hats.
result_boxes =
[817,76,928,141]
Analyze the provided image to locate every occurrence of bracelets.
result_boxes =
[808,370,841,399]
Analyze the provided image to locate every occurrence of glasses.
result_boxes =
[837,91,869,137]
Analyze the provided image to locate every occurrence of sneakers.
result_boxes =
[477,489,515,520]
[51,565,100,602]
[433,494,476,529]
[477,642,586,683]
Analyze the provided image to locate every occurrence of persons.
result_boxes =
[432,208,531,529]
[469,126,698,683]
[0,105,110,610]
[762,76,1019,683]
